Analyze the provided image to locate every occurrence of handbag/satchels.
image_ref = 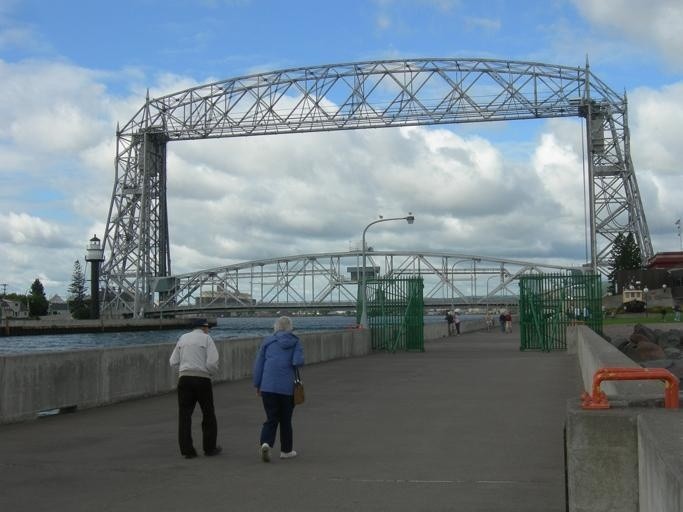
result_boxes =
[294,378,305,404]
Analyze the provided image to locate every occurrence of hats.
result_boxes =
[191,317,209,326]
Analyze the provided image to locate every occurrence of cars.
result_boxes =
[617,299,646,312]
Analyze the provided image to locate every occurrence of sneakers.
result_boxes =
[262,443,271,462]
[279,450,296,459]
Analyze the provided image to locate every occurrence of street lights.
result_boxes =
[635,281,640,290]
[643,287,648,319]
[451,258,480,317]
[358,214,414,330]
[630,284,634,290]
[662,284,666,322]
[485,273,507,317]
[506,282,520,315]
[567,296,574,299]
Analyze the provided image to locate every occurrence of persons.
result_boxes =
[569,304,589,321]
[444,311,454,337]
[499,312,505,331]
[252,316,304,463]
[661,307,666,322]
[169,317,223,458]
[453,309,461,336]
[674,301,681,321]
[503,312,512,337]
